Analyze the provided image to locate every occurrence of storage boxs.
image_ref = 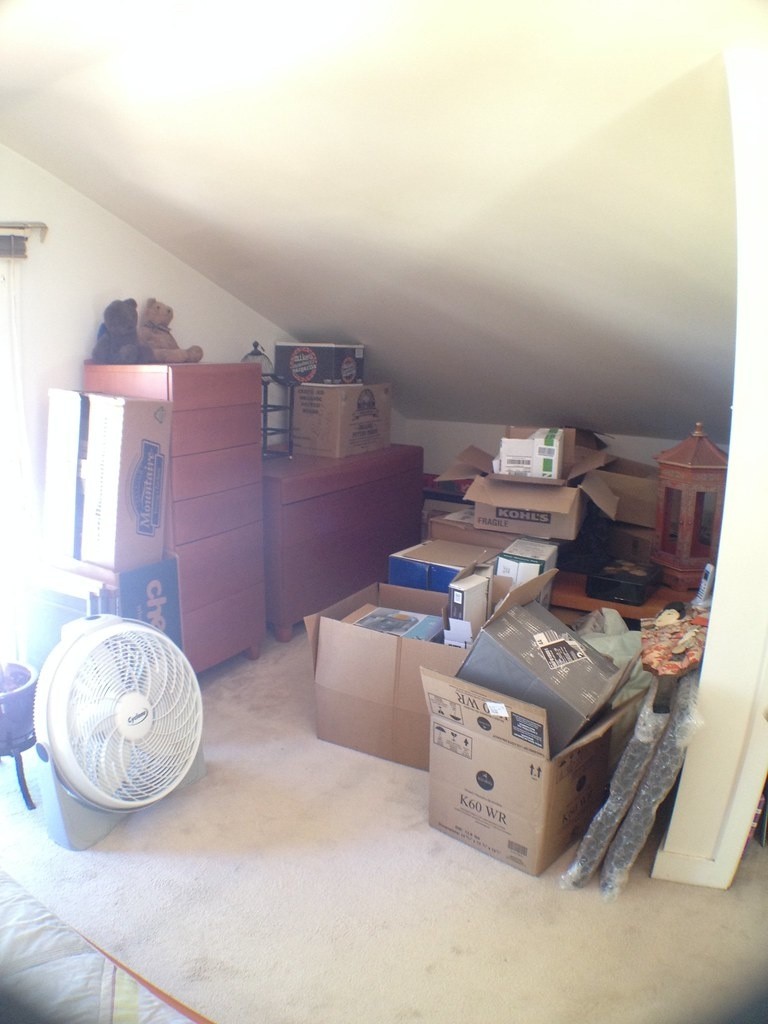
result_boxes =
[294,386,391,457]
[43,385,170,571]
[272,341,365,387]
[13,548,180,673]
[302,426,669,878]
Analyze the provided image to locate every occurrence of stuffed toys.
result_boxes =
[93,298,157,364]
[138,296,203,364]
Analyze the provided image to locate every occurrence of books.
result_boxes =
[741,786,768,856]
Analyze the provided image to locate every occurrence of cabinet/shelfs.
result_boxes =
[261,441,424,644]
[82,362,266,674]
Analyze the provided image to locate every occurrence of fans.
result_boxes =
[32,613,203,852]
